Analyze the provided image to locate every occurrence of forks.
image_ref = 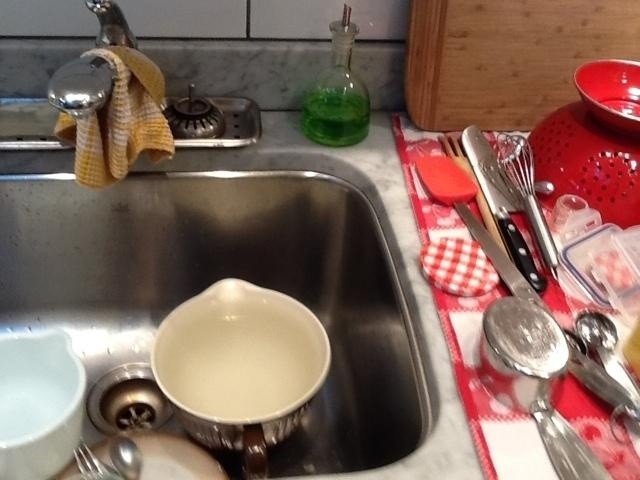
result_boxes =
[75,444,113,480]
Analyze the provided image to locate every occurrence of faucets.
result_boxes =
[47,0,136,119]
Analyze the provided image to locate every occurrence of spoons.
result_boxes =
[109,435,143,479]
[572,310,640,403]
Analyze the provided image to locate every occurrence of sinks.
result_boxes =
[0,147,439,480]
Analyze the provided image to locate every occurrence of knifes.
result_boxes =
[456,202,639,419]
[463,123,546,293]
[535,410,616,480]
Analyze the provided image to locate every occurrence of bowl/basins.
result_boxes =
[0,331,87,480]
[576,59,640,138]
[153,279,330,480]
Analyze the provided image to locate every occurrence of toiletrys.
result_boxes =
[298,4,372,149]
